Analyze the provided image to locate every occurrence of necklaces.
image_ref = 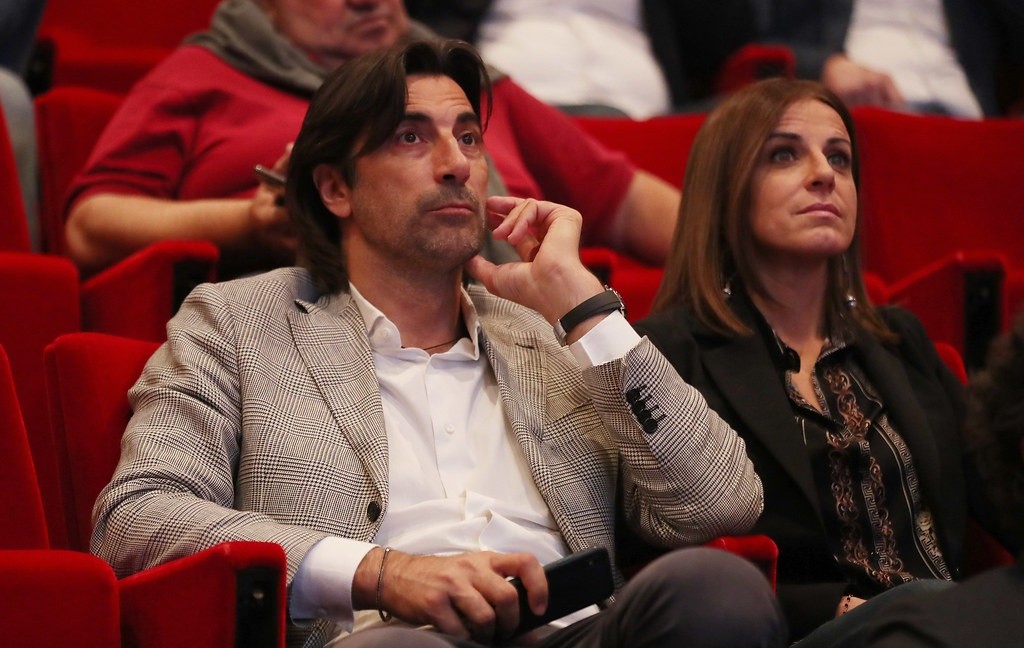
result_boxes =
[401,336,461,350]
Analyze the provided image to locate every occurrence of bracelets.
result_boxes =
[376,547,396,622]
[552,285,626,347]
[842,594,855,615]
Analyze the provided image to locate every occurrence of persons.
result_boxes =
[88,33,785,648]
[630,77,1024,648]
[0,0,1024,307]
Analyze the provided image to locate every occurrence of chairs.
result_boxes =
[0,0,1024,648]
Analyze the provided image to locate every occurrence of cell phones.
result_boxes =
[486,547,616,641]
[255,164,288,188]
